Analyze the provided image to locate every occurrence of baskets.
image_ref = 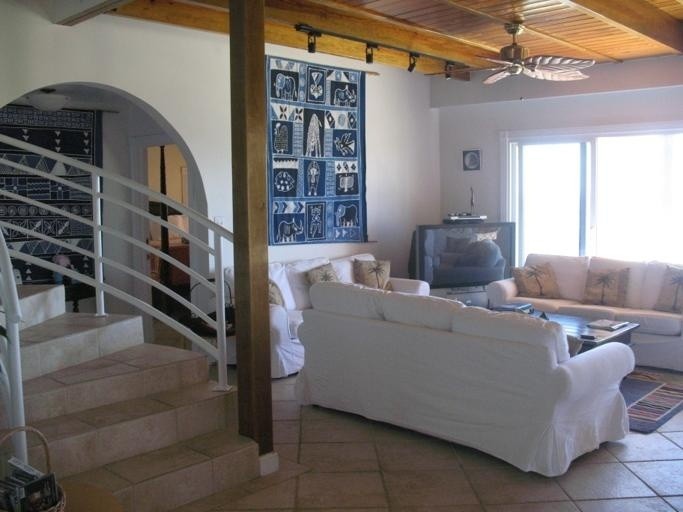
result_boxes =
[0,426,66,512]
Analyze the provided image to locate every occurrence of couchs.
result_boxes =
[487,254,683,372]
[297,285,635,477]
[225,254,431,380]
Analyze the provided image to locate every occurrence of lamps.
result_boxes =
[365,44,374,63]
[307,32,319,54]
[445,60,456,80]
[407,53,418,71]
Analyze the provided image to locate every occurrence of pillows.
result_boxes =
[582,268,628,307]
[653,266,683,313]
[512,264,561,298]
[304,264,339,284]
[352,261,390,290]
[268,283,283,305]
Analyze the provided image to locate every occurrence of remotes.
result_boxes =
[580,333,596,340]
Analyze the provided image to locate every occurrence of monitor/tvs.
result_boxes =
[407,222,517,289]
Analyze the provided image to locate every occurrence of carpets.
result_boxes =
[619,372,683,433]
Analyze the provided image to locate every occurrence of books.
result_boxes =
[586,319,630,331]
[0,455,59,512]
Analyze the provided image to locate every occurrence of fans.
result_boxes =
[424,23,595,86]
